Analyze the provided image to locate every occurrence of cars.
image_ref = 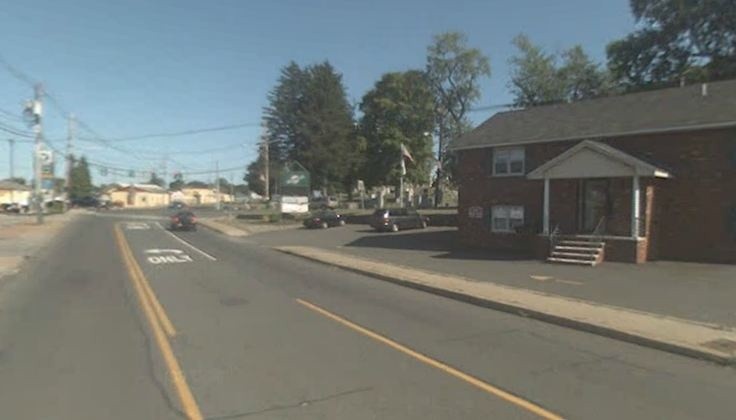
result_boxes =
[0,194,112,215]
[169,201,184,209]
[303,210,348,229]
[169,210,197,232]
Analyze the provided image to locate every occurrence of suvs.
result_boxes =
[369,207,431,232]
